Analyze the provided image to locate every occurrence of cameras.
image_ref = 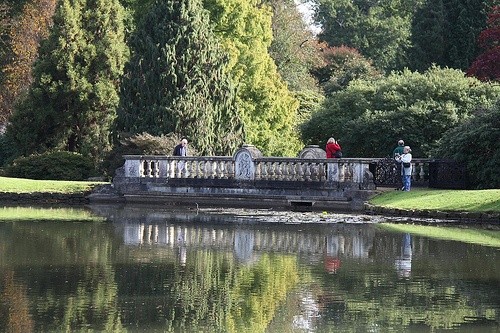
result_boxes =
[395,153,399,156]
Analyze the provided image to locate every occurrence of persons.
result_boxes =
[172,138,188,178]
[393,140,405,191]
[395,145,412,192]
[326,137,341,181]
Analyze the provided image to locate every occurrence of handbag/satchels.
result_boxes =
[332,150,342,158]
[403,162,410,168]
